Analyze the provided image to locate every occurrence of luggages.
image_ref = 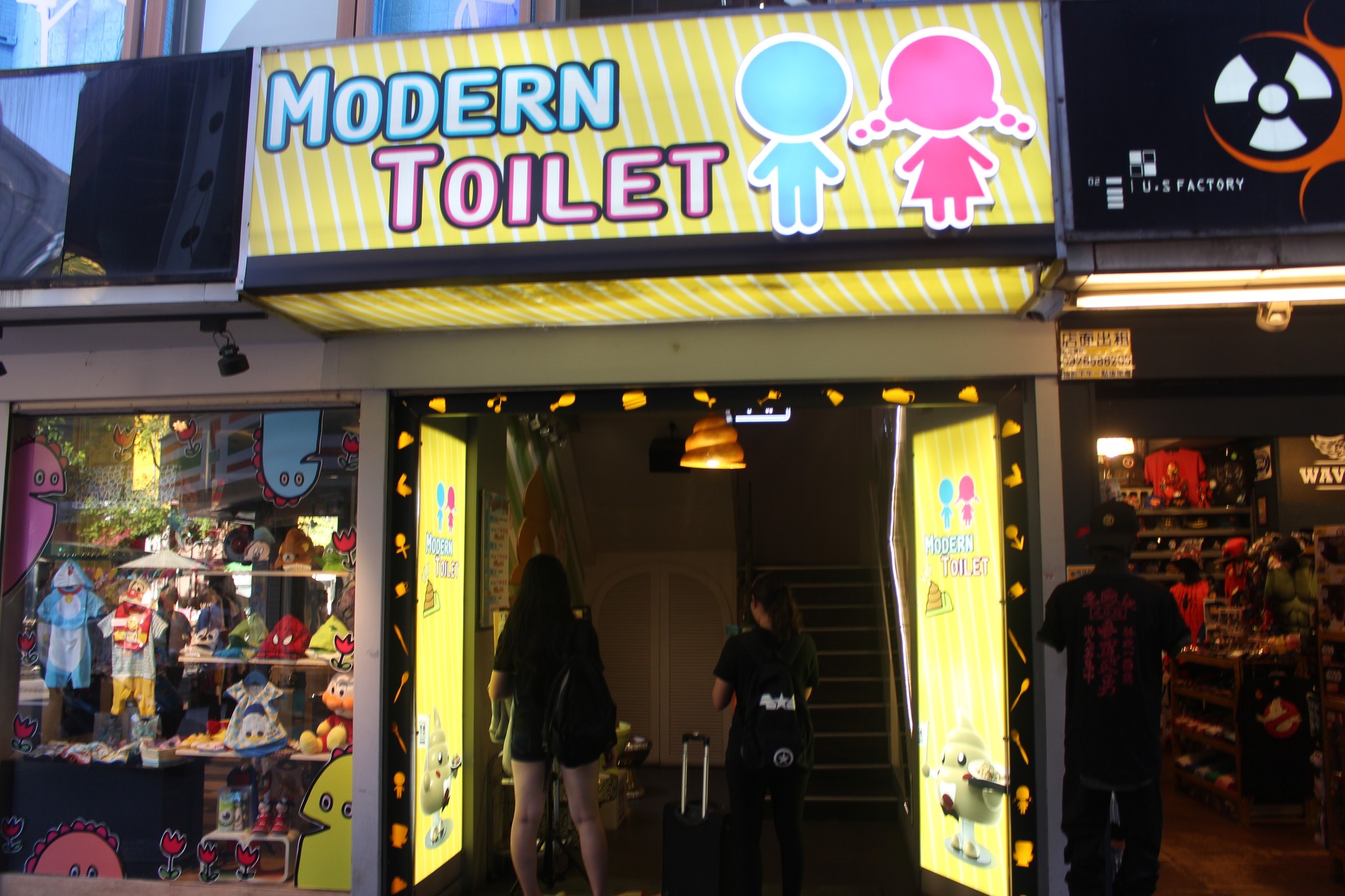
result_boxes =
[662,732,734,896]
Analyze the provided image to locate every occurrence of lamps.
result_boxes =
[199,317,249,376]
[678,386,747,472]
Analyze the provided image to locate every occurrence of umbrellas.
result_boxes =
[116,550,211,586]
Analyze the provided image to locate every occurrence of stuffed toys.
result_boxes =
[299,671,354,757]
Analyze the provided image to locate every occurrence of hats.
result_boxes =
[1086,500,1137,547]
[1127,497,1251,574]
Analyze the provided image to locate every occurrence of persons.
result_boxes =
[60,622,106,742]
[1037,499,1193,896]
[713,573,819,896]
[157,573,250,736]
[308,578,354,628]
[488,554,618,896]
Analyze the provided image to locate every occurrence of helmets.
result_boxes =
[1262,701,1301,739]
[1169,545,1203,572]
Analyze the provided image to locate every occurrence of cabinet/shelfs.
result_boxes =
[1129,489,1257,581]
[175,570,354,883]
[1168,651,1316,828]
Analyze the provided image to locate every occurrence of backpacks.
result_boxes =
[738,630,811,777]
[544,610,617,769]
